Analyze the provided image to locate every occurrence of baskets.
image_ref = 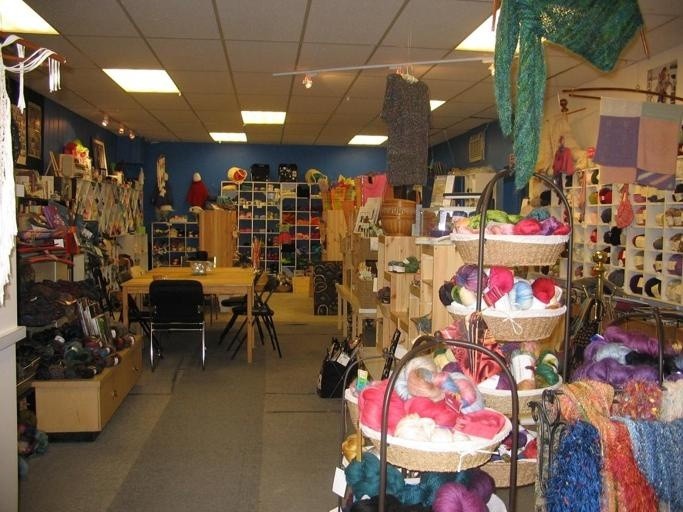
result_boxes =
[478,375,563,418]
[449,231,569,268]
[345,390,511,474]
[480,460,537,488]
[449,306,567,342]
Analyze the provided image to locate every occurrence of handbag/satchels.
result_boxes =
[318,337,359,398]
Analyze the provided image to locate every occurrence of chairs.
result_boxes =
[116,265,283,373]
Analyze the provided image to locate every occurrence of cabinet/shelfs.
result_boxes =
[73,174,143,240]
[545,155,682,312]
[199,210,238,268]
[215,179,322,276]
[378,229,453,368]
[31,334,143,442]
[149,220,199,269]
[331,234,379,345]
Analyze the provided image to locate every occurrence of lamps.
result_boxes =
[267,53,498,90]
[98,109,139,141]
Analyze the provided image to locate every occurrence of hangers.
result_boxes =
[397,63,418,82]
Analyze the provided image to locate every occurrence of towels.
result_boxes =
[591,92,682,193]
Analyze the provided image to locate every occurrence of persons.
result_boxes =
[151,172,174,216]
[656,67,666,103]
[186,171,208,209]
[668,74,677,101]
